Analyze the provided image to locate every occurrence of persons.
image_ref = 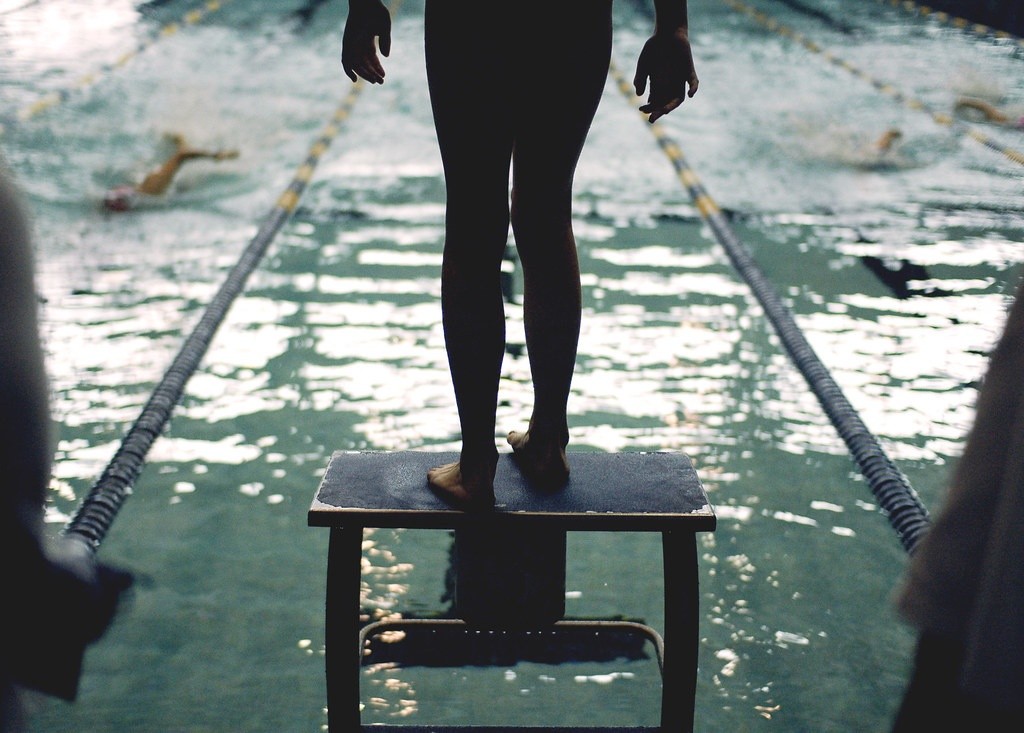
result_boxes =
[342,0,699,510]
[887,269,1023,733]
[103,129,242,216]
[1,175,56,733]
[849,94,1024,175]
